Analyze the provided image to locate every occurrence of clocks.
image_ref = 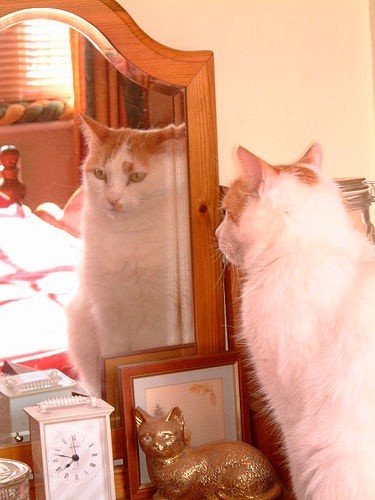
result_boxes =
[21,394,118,500]
[1,368,79,448]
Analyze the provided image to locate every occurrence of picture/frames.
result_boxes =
[102,341,199,427]
[115,349,254,500]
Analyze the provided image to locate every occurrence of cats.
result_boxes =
[64,112,195,400]
[132,409,284,495]
[208,141,374,500]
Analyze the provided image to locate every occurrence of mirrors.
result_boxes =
[1,0,227,475]
